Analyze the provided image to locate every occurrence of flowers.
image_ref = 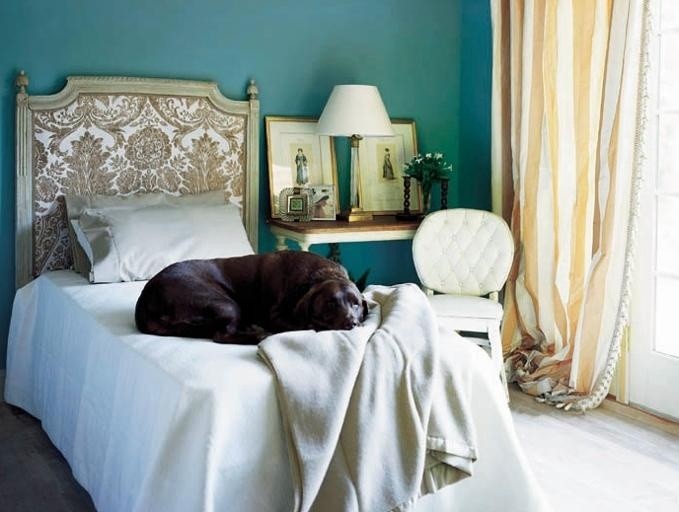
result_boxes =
[403,150,452,206]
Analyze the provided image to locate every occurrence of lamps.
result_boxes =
[313,84,397,222]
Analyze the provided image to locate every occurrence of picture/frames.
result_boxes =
[307,183,337,222]
[264,114,339,219]
[357,117,424,216]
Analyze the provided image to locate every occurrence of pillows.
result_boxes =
[70,203,255,283]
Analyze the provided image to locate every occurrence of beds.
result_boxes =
[0,69,545,512]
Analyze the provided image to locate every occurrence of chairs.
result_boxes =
[412,209,516,407]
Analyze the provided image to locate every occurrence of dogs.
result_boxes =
[134,250,369,345]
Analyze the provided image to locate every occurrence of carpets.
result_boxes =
[0,400,98,512]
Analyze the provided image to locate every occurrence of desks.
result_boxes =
[269,216,418,296]
